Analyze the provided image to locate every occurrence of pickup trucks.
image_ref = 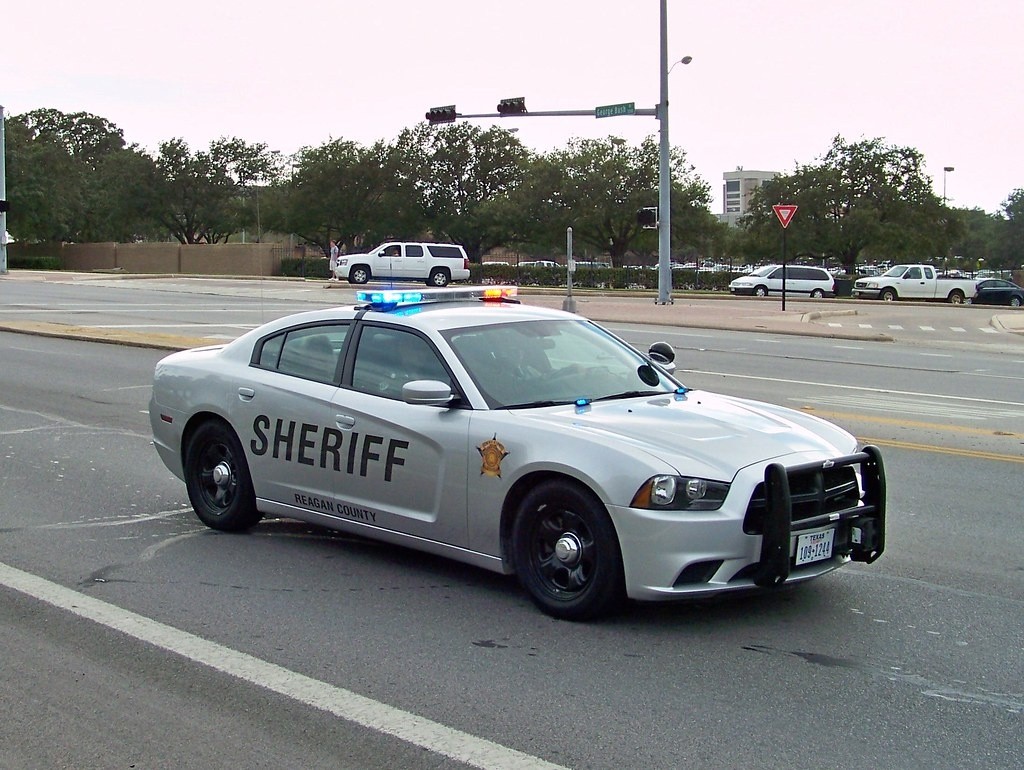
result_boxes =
[851,264,978,305]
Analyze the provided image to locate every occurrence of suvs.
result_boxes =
[333,243,471,285]
[728,265,837,296]
[512,260,561,268]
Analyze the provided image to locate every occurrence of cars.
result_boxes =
[622,261,754,273]
[148,286,887,623]
[963,277,1024,306]
[828,264,962,278]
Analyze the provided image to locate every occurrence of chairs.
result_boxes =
[294,335,337,379]
[365,334,404,368]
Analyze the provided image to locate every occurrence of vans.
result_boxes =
[482,262,509,266]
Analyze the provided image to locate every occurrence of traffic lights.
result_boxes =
[497,96,526,114]
[425,105,456,125]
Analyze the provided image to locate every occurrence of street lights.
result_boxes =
[943,167,955,206]
[653,56,692,306]
